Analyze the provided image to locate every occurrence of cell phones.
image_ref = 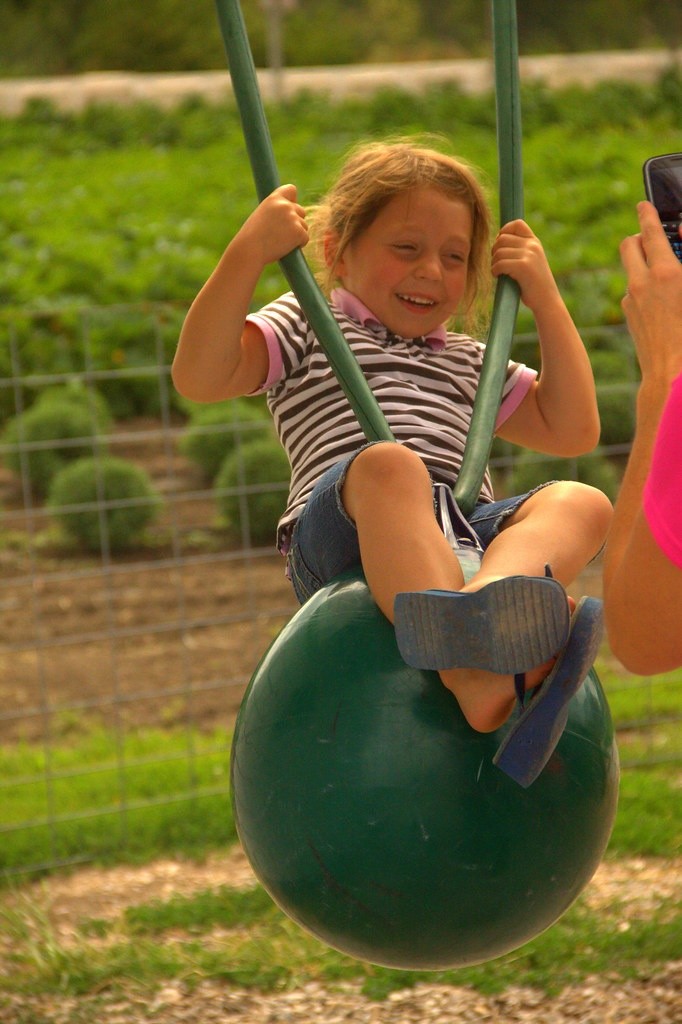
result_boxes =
[643,153,682,264]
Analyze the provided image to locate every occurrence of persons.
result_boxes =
[170,130,615,790]
[600,199,681,675]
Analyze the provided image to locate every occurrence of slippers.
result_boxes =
[391,576,569,673]
[491,595,603,791]
